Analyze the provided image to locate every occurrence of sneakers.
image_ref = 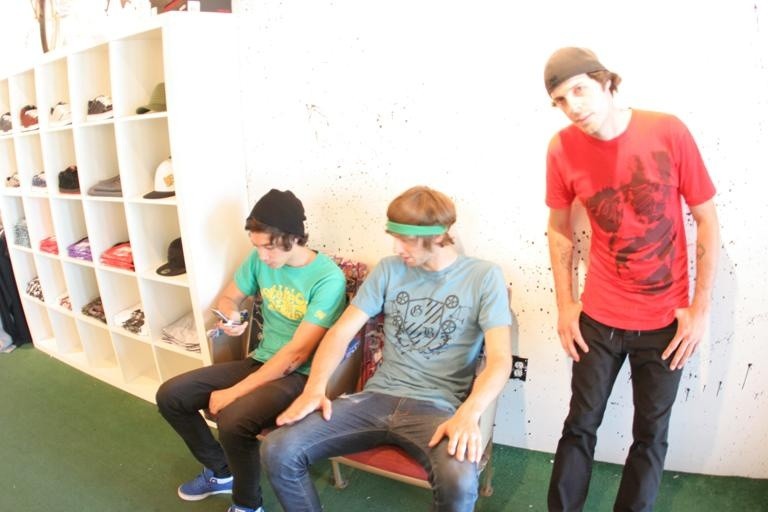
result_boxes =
[228,502,265,512]
[177,468,234,502]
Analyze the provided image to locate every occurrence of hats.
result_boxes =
[0,83,188,277]
[544,47,609,96]
[252,188,305,239]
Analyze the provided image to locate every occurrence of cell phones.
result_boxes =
[211,308,243,326]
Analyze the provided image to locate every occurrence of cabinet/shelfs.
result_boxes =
[1,8,236,431]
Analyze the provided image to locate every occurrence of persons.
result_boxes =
[157,189,346,511]
[546,47,721,511]
[260,186,513,511]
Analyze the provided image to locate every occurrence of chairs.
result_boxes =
[328,283,512,498]
[206,255,369,444]
